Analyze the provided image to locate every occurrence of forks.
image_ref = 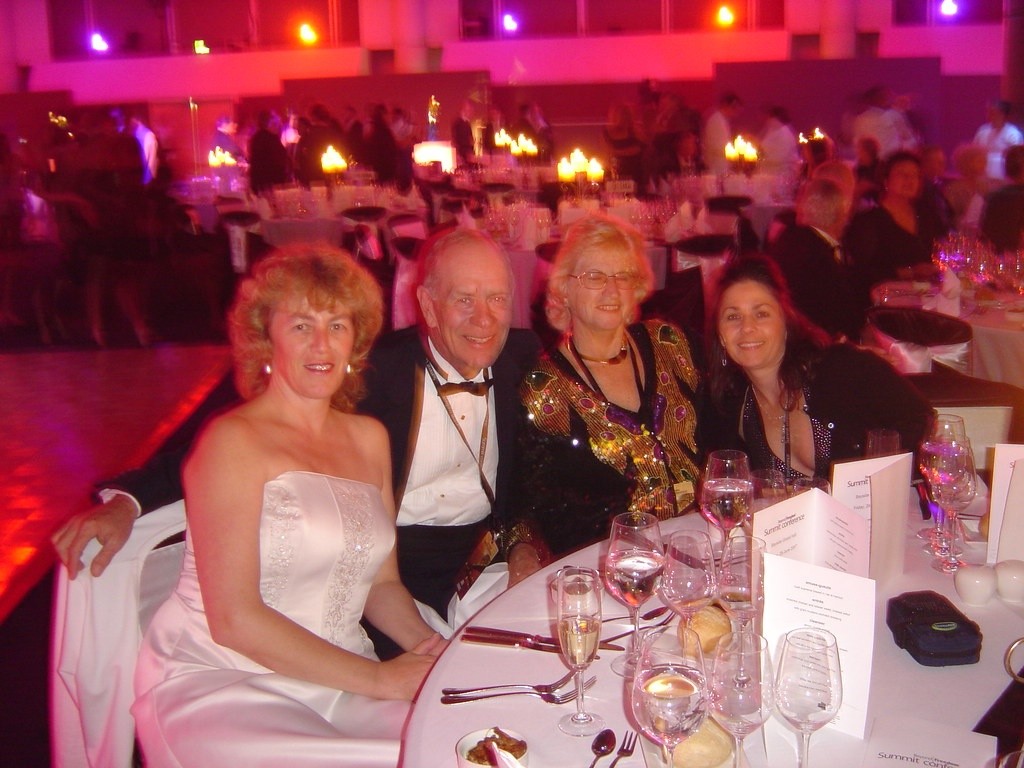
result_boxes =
[609,730,639,768]
[440,668,598,706]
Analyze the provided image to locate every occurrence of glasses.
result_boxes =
[567,271,639,290]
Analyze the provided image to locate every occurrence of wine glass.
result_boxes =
[604,511,665,680]
[713,535,766,694]
[920,414,979,573]
[929,229,1024,316]
[658,529,718,656]
[556,566,606,737]
[629,626,710,768]
[707,631,776,768]
[775,627,844,768]
[698,449,832,592]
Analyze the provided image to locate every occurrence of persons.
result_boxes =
[0,108,199,348]
[207,100,553,194]
[134,246,455,768]
[600,77,1024,362]
[705,254,937,490]
[518,220,710,555]
[51,230,540,659]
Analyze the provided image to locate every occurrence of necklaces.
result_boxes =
[750,382,793,445]
[563,327,629,366]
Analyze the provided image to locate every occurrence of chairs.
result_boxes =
[50,500,187,768]
[857,306,973,409]
[226,184,756,329]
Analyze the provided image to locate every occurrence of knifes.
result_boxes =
[459,625,625,660]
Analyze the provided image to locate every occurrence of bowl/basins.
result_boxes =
[455,728,530,768]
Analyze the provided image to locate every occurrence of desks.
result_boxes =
[401,484,1024,768]
[391,241,667,329]
[890,259,1024,394]
[231,189,419,256]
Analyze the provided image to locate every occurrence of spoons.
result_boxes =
[588,729,617,768]
[601,606,669,624]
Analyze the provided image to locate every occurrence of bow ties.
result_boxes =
[437,381,488,397]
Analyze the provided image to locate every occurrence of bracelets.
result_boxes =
[502,522,533,561]
[805,323,818,340]
